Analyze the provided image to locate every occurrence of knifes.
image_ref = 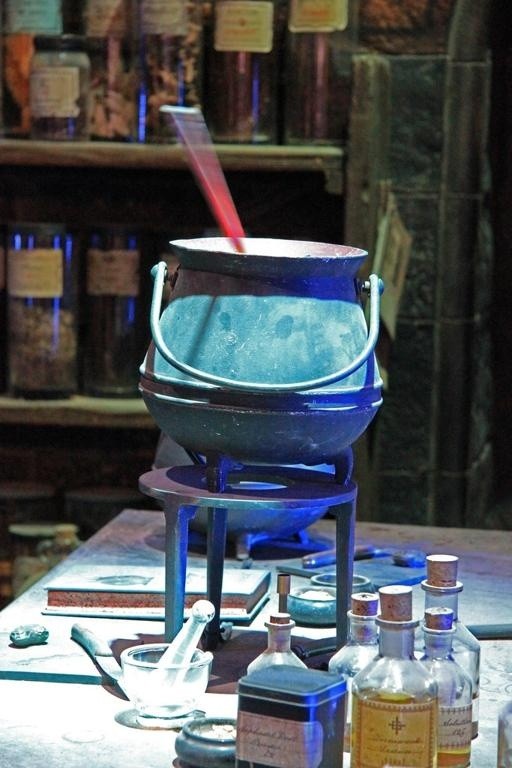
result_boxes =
[69,627,126,701]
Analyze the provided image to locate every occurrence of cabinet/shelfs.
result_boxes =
[1,41,390,609]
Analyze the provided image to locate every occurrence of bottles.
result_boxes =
[76,0,138,143]
[1,524,85,591]
[246,612,308,682]
[0,0,73,141]
[497,684,512,767]
[138,1,209,144]
[1,213,147,401]
[283,0,353,147]
[209,1,282,146]
[327,553,482,768]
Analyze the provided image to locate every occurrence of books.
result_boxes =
[40,565,272,621]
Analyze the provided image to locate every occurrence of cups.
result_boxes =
[122,643,212,729]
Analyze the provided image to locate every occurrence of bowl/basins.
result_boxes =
[173,716,237,766]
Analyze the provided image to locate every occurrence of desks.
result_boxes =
[1,507,511,768]
